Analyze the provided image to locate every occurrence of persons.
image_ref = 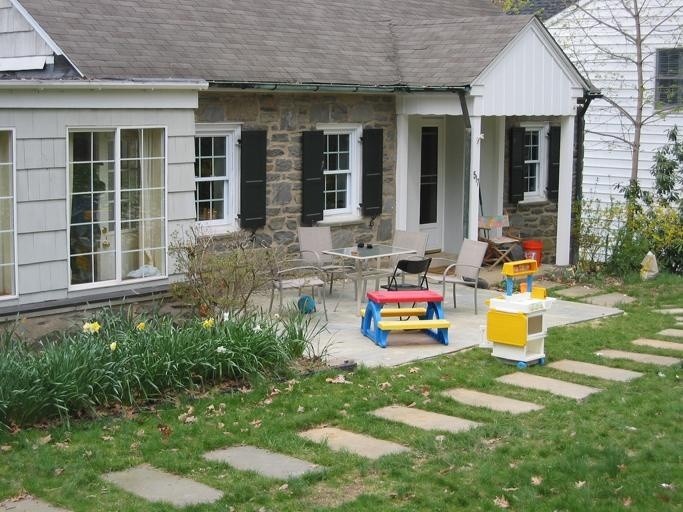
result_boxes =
[70,173,106,286]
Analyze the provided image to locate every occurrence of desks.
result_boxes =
[364,290,445,346]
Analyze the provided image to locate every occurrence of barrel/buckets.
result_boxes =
[298,294,315,315]
[522,240,544,267]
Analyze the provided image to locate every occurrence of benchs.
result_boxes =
[358,306,452,345]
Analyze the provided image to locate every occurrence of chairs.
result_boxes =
[262,214,519,321]
